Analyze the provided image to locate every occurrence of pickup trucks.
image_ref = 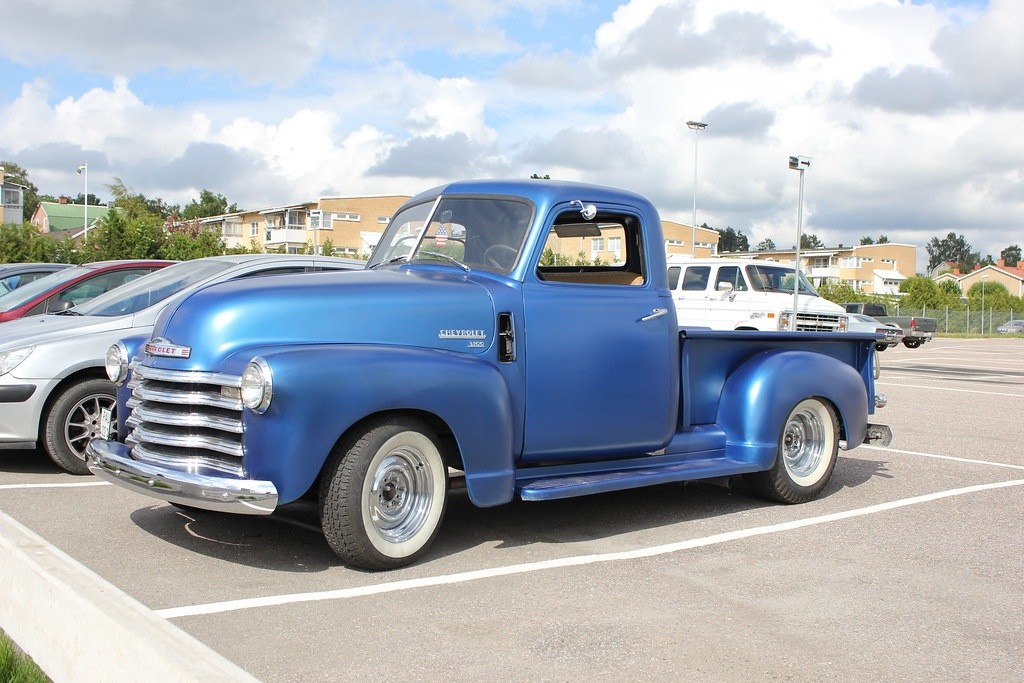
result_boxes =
[87,178,896,572]
[838,302,939,352]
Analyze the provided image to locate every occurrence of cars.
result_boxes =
[1,253,367,478]
[1,263,73,297]
[843,311,905,352]
[996,319,1024,336]
[1,259,178,322]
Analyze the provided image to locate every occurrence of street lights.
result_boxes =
[685,121,709,256]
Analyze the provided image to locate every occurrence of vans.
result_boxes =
[664,258,848,333]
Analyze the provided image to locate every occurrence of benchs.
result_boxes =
[543,272,644,286]
